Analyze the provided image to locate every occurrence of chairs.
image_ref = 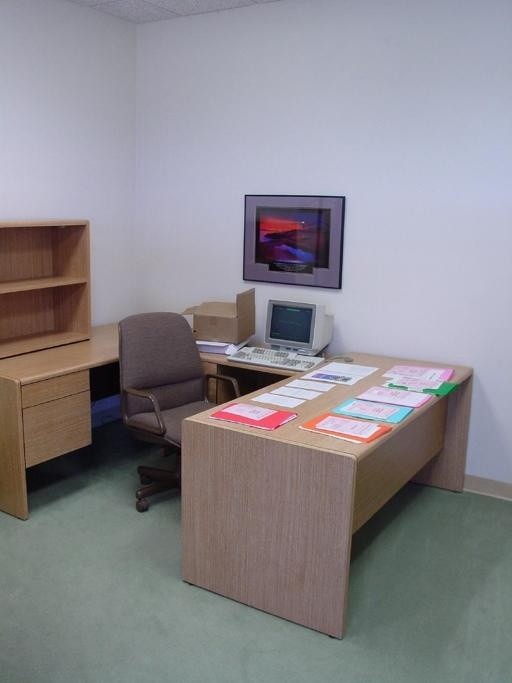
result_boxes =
[118,312,240,513]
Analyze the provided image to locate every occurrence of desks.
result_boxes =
[0,323,473,640]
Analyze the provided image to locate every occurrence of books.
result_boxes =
[299,359,381,386]
[387,373,461,398]
[210,399,299,431]
[353,385,434,410]
[381,363,457,381]
[328,397,415,424]
[298,411,396,445]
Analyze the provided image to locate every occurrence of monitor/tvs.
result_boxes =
[264,298,334,356]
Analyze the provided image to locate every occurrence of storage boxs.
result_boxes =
[181,287,255,344]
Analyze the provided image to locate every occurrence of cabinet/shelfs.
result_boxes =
[0,220,92,359]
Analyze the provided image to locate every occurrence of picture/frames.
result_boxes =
[243,195,345,290]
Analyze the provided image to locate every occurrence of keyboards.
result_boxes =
[227,346,326,372]
